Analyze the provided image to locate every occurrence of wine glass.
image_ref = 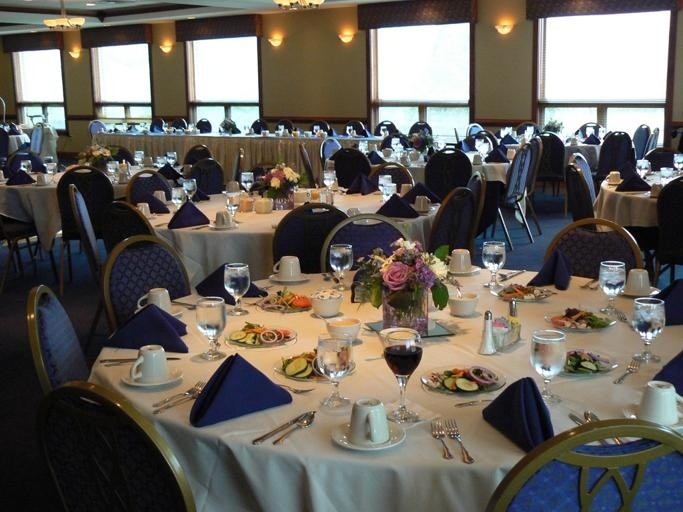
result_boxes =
[221,262,252,317]
[382,329,423,426]
[629,299,664,366]
[596,260,624,315]
[329,243,353,295]
[19,150,177,186]
[499,124,607,147]
[312,332,352,409]
[529,327,571,403]
[109,119,389,139]
[194,295,227,360]
[482,241,505,291]
[356,138,519,166]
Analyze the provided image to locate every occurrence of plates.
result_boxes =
[223,327,300,347]
[554,348,617,379]
[271,354,357,378]
[266,274,311,283]
[622,286,660,299]
[117,369,182,387]
[488,287,555,303]
[130,304,185,318]
[253,297,310,313]
[541,312,616,335]
[621,401,682,432]
[420,365,507,395]
[443,265,480,276]
[329,420,405,453]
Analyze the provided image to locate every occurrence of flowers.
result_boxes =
[368,238,449,290]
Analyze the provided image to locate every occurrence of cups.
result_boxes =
[605,152,683,198]
[627,268,651,294]
[136,287,172,314]
[130,344,168,383]
[272,255,301,280]
[347,397,391,446]
[636,380,682,426]
[135,170,431,230]
[449,249,468,271]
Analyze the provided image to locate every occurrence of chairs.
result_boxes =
[485,419,682,512]
[26,283,90,395]
[1,117,683,343]
[38,381,196,511]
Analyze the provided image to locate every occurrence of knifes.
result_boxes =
[99,356,181,365]
[250,410,320,445]
[169,300,196,310]
[500,270,525,282]
[321,271,339,284]
[568,413,608,447]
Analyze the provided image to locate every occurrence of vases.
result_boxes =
[382,289,431,338]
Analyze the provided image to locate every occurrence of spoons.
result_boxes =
[273,412,316,446]
[583,409,622,445]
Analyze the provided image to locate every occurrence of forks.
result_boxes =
[277,382,315,394]
[447,414,475,465]
[499,270,517,278]
[615,310,635,331]
[149,379,208,417]
[614,360,643,388]
[578,278,599,291]
[428,417,452,463]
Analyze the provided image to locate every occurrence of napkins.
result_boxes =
[527,250,572,292]
[104,304,190,353]
[194,263,267,305]
[650,278,682,326]
[481,377,555,453]
[653,351,683,397]
[189,352,293,428]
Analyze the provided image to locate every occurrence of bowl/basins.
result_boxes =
[312,296,342,319]
[324,316,361,345]
[378,326,419,350]
[446,292,480,319]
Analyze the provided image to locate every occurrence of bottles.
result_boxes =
[477,312,497,355]
[507,300,516,317]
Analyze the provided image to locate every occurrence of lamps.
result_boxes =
[42,0,85,31]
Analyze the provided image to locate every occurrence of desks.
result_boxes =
[95,268,681,472]
